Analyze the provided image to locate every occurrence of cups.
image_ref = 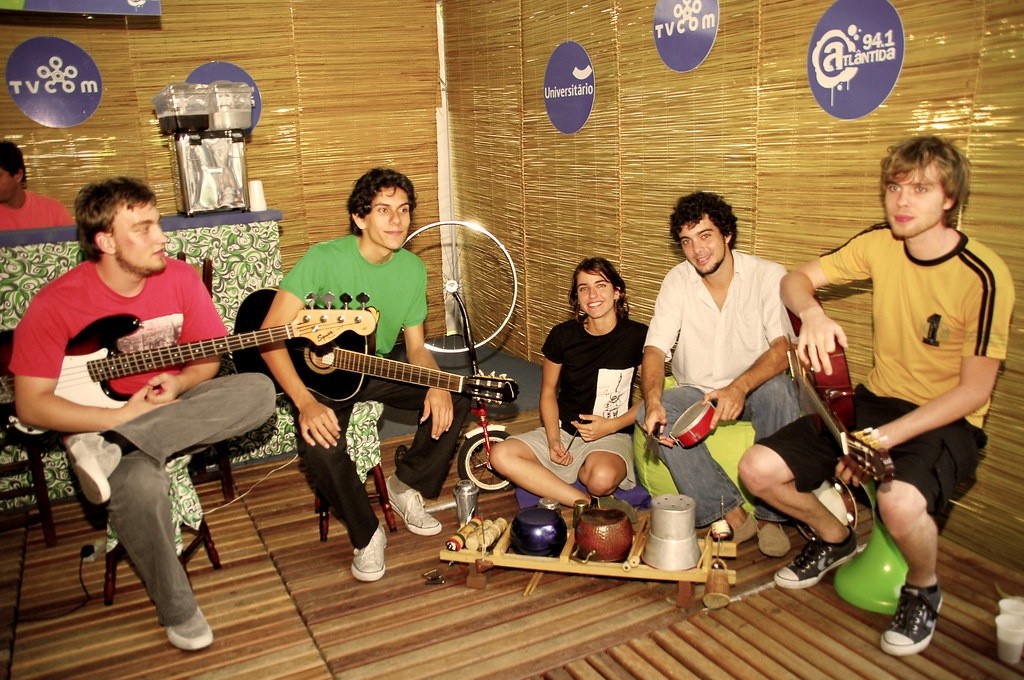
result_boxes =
[995,614,1024,666]
[998,598,1024,614]
[249,180,266,212]
[573,499,588,526]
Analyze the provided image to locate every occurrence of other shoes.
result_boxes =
[753,515,790,557]
[166,605,213,650]
[729,511,757,545]
[65,431,121,503]
[589,495,639,524]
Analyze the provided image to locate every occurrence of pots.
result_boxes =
[571,510,632,566]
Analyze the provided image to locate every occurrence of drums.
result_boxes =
[670,400,716,447]
[796,476,857,543]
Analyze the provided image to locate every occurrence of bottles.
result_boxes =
[453,480,481,526]
[538,498,560,510]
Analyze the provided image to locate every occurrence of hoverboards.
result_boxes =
[401,220,519,495]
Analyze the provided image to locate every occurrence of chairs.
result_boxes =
[316,416,398,543]
[0,328,58,546]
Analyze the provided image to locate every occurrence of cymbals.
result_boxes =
[810,477,854,541]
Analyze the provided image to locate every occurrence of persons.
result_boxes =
[0,139,75,234]
[255,168,471,582]
[634,191,799,556]
[9,177,277,651]
[740,133,1015,656]
[491,255,672,526]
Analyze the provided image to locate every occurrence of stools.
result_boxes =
[75,476,223,605]
[834,478,909,616]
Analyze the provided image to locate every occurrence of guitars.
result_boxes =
[783,293,895,485]
[0,292,377,452]
[232,286,521,405]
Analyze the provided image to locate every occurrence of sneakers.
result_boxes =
[775,527,857,588]
[385,476,442,536]
[351,522,387,582]
[880,585,942,657]
